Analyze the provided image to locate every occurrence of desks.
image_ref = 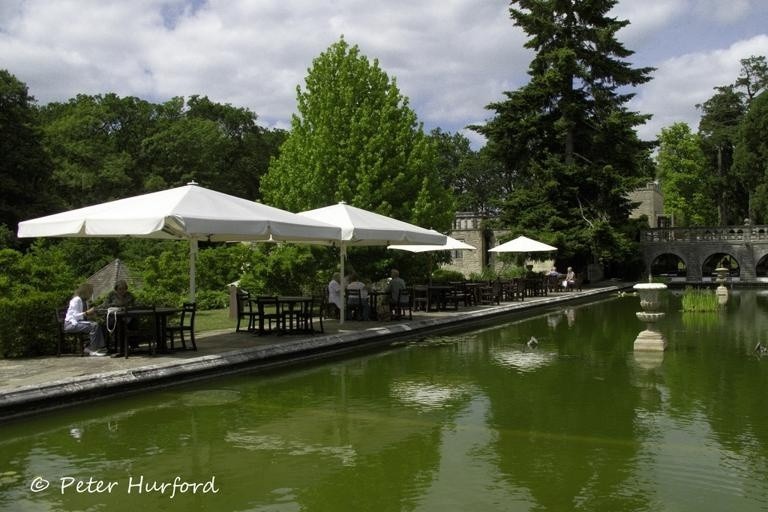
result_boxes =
[91,305,180,359]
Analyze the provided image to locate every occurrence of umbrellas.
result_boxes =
[18,180,343,311]
[384,225,476,285]
[225,198,448,284]
[386,374,481,412]
[488,234,559,279]
[488,336,558,375]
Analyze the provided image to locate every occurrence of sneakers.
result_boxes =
[83,347,91,353]
[89,350,106,357]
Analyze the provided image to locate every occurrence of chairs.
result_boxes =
[166,301,197,351]
[123,302,159,359]
[232,270,583,339]
[54,304,95,356]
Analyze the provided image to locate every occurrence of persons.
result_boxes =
[564,266,577,291]
[328,272,351,321]
[103,280,138,356]
[383,269,410,322]
[545,266,561,293]
[67,425,86,445]
[63,283,108,356]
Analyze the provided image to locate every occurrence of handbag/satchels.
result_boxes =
[105,306,121,332]
